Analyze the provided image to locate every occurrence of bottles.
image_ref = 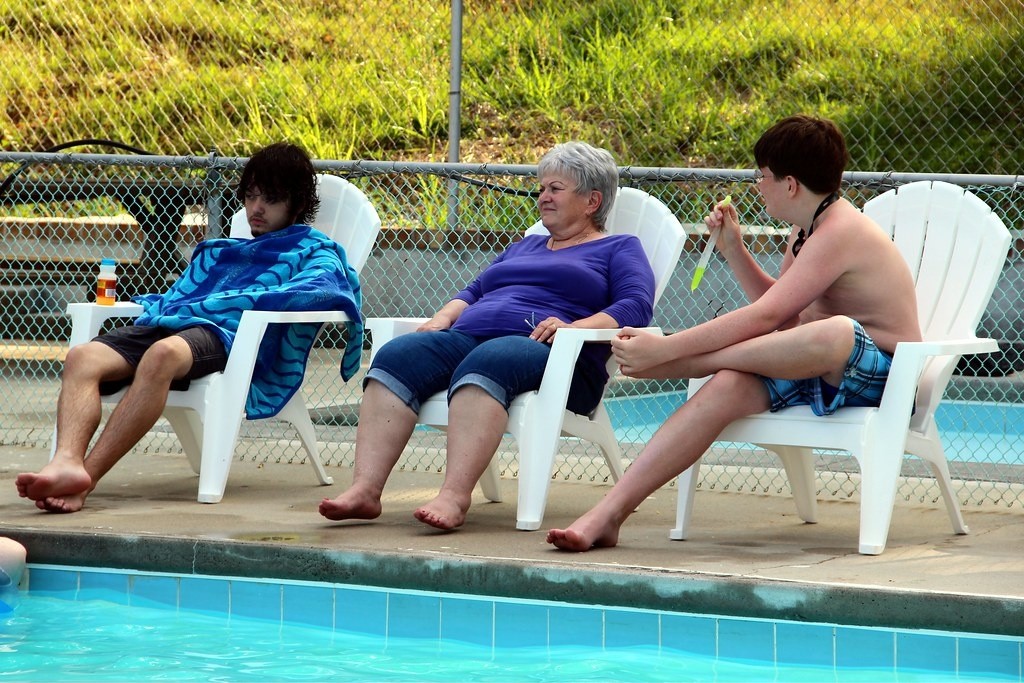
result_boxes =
[96,259,116,305]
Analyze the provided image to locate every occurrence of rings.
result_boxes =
[552,324,557,330]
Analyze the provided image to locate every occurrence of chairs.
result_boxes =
[364,187,685,530]
[48,173,382,502]
[671,180,1013,554]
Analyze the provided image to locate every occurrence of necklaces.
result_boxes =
[551,235,588,250]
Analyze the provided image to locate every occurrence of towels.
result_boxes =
[127,221,365,422]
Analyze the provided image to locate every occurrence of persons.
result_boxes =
[15,141,338,512]
[547,115,921,552]
[318,141,655,529]
